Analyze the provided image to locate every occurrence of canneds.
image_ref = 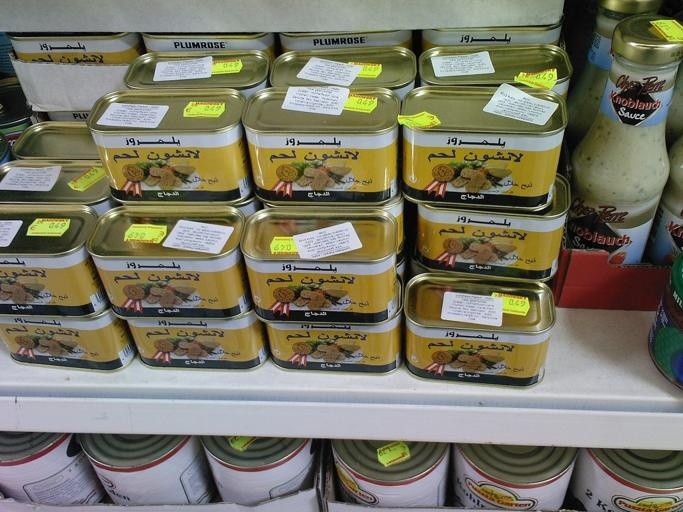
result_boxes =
[263,192,404,255]
[0,431,115,504]
[270,46,417,103]
[122,50,270,99]
[397,251,409,284]
[422,15,564,53]
[266,305,403,374]
[419,44,572,101]
[447,442,579,512]
[241,87,400,205]
[142,32,276,64]
[47,111,91,121]
[78,433,222,504]
[3,307,138,373]
[279,30,414,54]
[1,132,11,165]
[0,205,109,318]
[647,251,683,392]
[240,207,396,323]
[123,195,259,220]
[4,31,147,112]
[0,13,572,393]
[404,272,555,390]
[1,85,48,148]
[126,305,268,371]
[562,448,683,512]
[11,120,101,160]
[416,172,571,278]
[86,88,254,204]
[411,258,557,289]
[86,206,252,320]
[198,435,321,507]
[401,85,567,211]
[1,160,113,218]
[330,439,451,507]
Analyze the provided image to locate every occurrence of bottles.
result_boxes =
[566,0,683,262]
